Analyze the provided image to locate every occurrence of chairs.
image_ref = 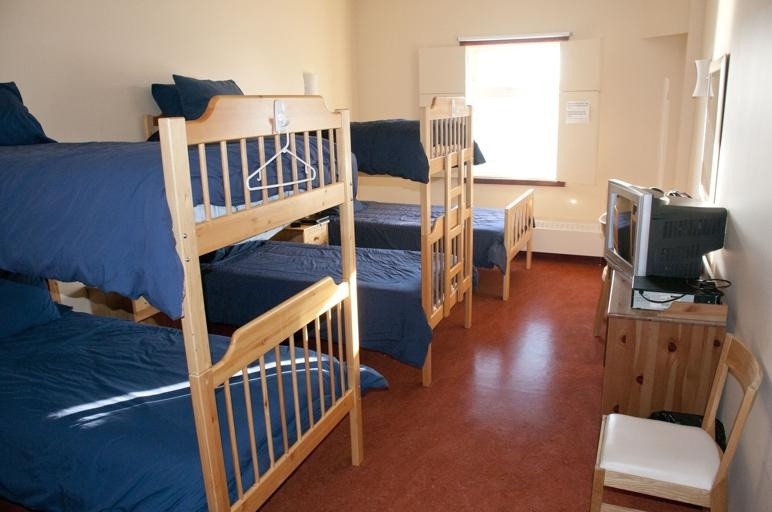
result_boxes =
[589,332,763,511]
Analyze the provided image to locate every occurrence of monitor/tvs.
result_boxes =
[603,179,727,282]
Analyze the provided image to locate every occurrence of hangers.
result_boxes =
[242,120,320,193]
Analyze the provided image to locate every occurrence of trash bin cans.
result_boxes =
[649,410,727,455]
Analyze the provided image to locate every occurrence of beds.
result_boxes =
[1,104,355,319]
[2,274,368,509]
[256,98,483,204]
[178,204,482,390]
[279,186,536,303]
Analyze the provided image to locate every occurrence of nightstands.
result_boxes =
[273,217,335,247]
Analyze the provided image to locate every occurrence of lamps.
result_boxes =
[689,58,711,99]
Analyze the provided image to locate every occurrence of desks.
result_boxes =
[599,269,730,419]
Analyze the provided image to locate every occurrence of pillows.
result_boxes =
[4,276,63,335]
[0,78,58,147]
[152,73,245,121]
